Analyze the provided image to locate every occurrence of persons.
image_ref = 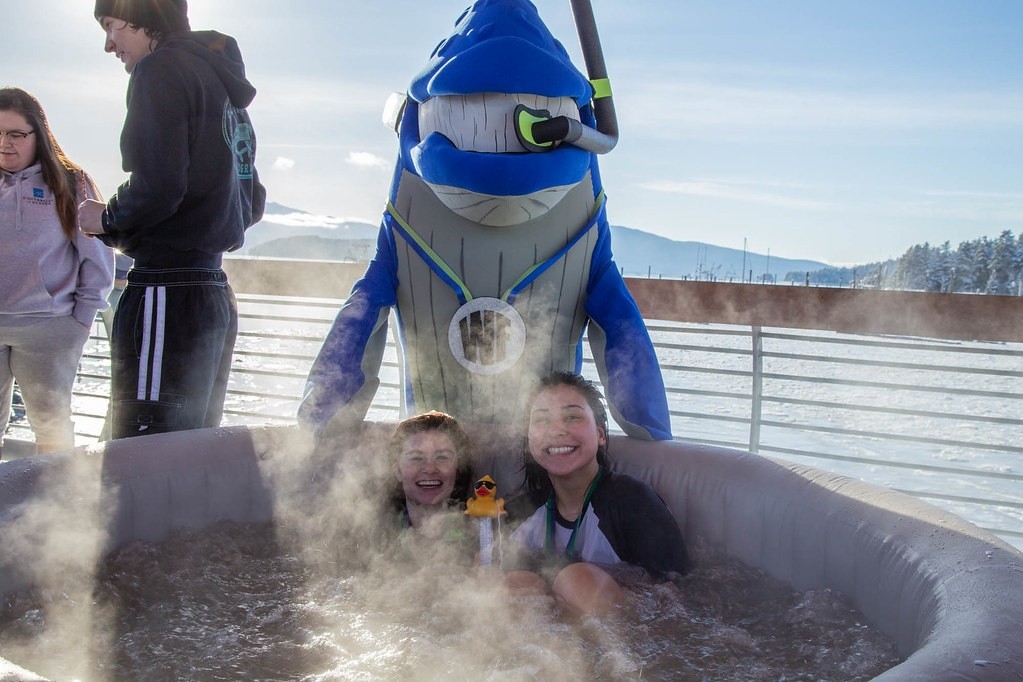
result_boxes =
[350,410,481,568]
[493,370,689,611]
[76,0,266,441]
[0,88,116,460]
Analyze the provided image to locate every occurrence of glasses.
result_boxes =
[0,129,37,146]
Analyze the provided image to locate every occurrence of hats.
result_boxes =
[94,0,190,34]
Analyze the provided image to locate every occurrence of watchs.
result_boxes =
[102,208,116,233]
